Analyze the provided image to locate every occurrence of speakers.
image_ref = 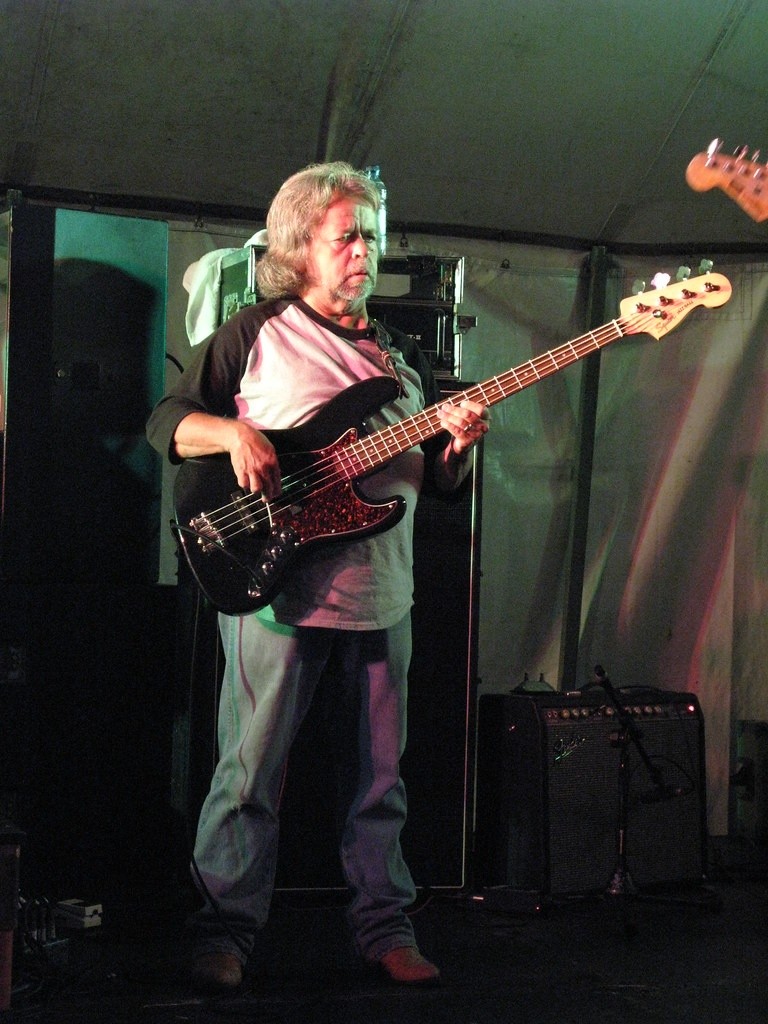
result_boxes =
[168,380,485,899]
[477,689,708,896]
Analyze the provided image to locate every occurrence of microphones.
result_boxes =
[640,788,689,806]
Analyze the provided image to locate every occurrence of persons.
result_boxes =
[145,160,490,994]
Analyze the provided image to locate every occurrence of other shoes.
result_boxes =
[374,946,440,987]
[193,945,243,992]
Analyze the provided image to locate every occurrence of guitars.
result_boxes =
[173,258,733,617]
[685,137,768,224]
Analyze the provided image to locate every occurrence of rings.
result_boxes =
[463,423,475,433]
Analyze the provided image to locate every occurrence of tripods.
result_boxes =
[540,664,725,949]
